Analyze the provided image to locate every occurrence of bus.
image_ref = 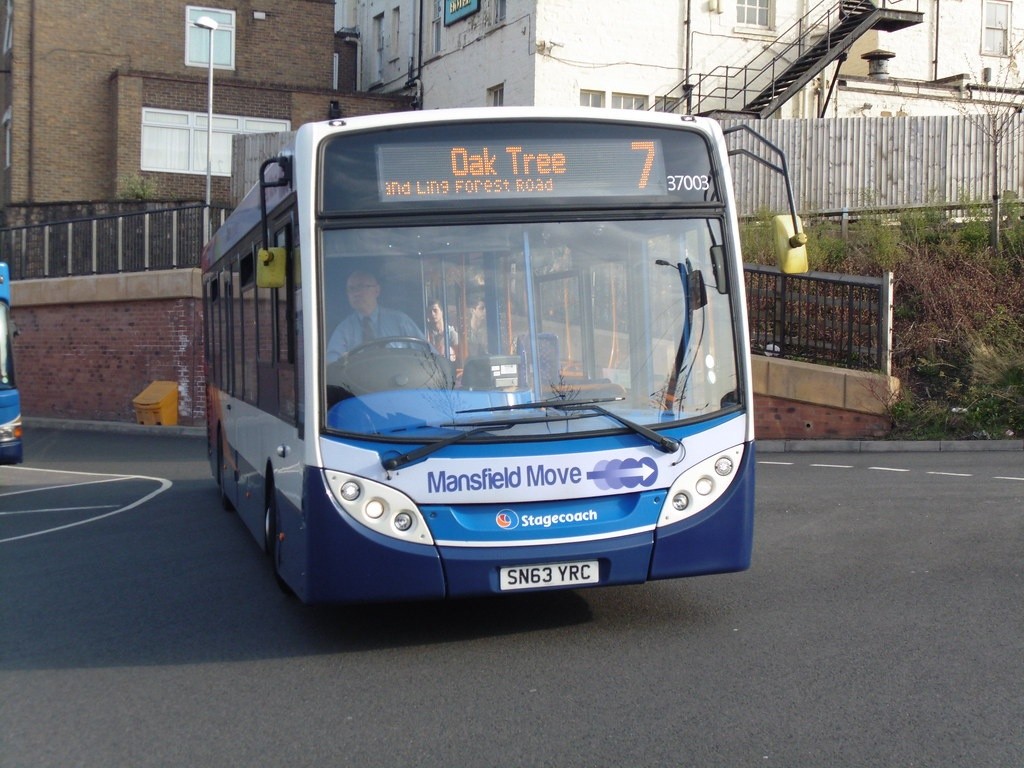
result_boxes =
[0,263,23,463]
[202,106,809,609]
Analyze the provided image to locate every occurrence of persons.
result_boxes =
[425,301,458,362]
[466,298,487,354]
[326,271,441,369]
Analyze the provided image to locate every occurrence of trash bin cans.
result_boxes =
[132,380,178,426]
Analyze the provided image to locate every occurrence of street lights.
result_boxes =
[194,15,219,250]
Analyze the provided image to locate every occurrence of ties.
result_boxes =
[361,317,376,350]
